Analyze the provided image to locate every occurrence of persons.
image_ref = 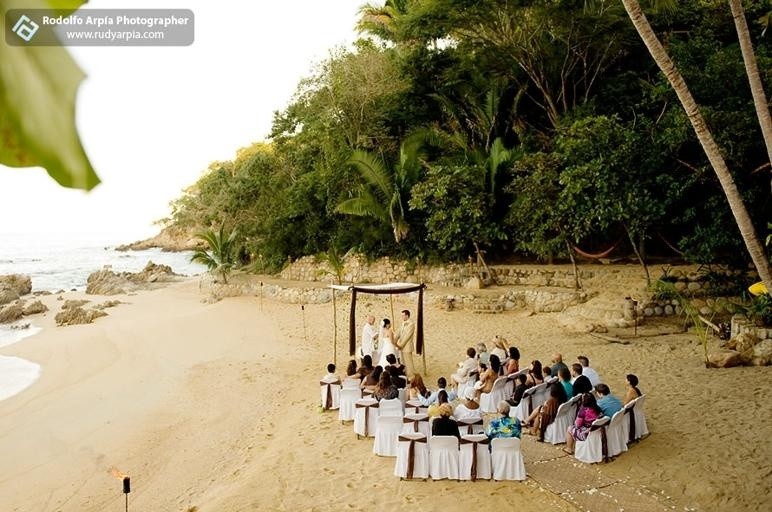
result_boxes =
[360,314,379,360]
[321,336,646,455]
[394,308,415,380]
[378,318,400,372]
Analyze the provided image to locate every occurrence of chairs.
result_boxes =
[456,357,652,465]
[320,375,527,483]
